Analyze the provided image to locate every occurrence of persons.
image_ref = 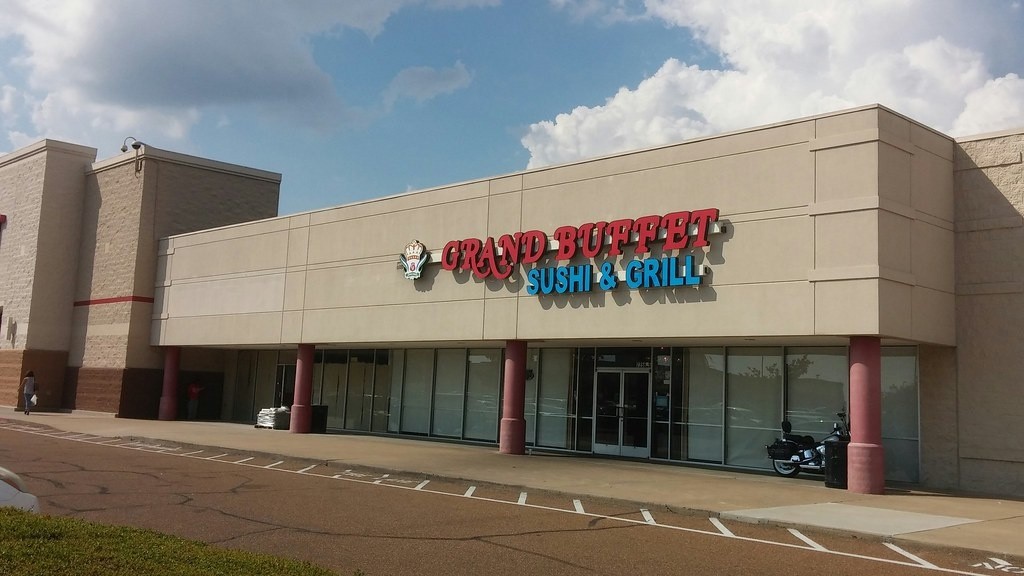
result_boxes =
[17,370,38,415]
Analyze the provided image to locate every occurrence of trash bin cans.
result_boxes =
[824,440,850,488]
[311,404,329,433]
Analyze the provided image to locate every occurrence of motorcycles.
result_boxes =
[765,405,850,478]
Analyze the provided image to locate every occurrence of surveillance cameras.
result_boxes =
[121,145,128,152]
[131,141,141,149]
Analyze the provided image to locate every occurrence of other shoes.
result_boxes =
[25,411,27,414]
[27,412,29,415]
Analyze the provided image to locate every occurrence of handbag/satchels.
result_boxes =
[30,395,39,406]
